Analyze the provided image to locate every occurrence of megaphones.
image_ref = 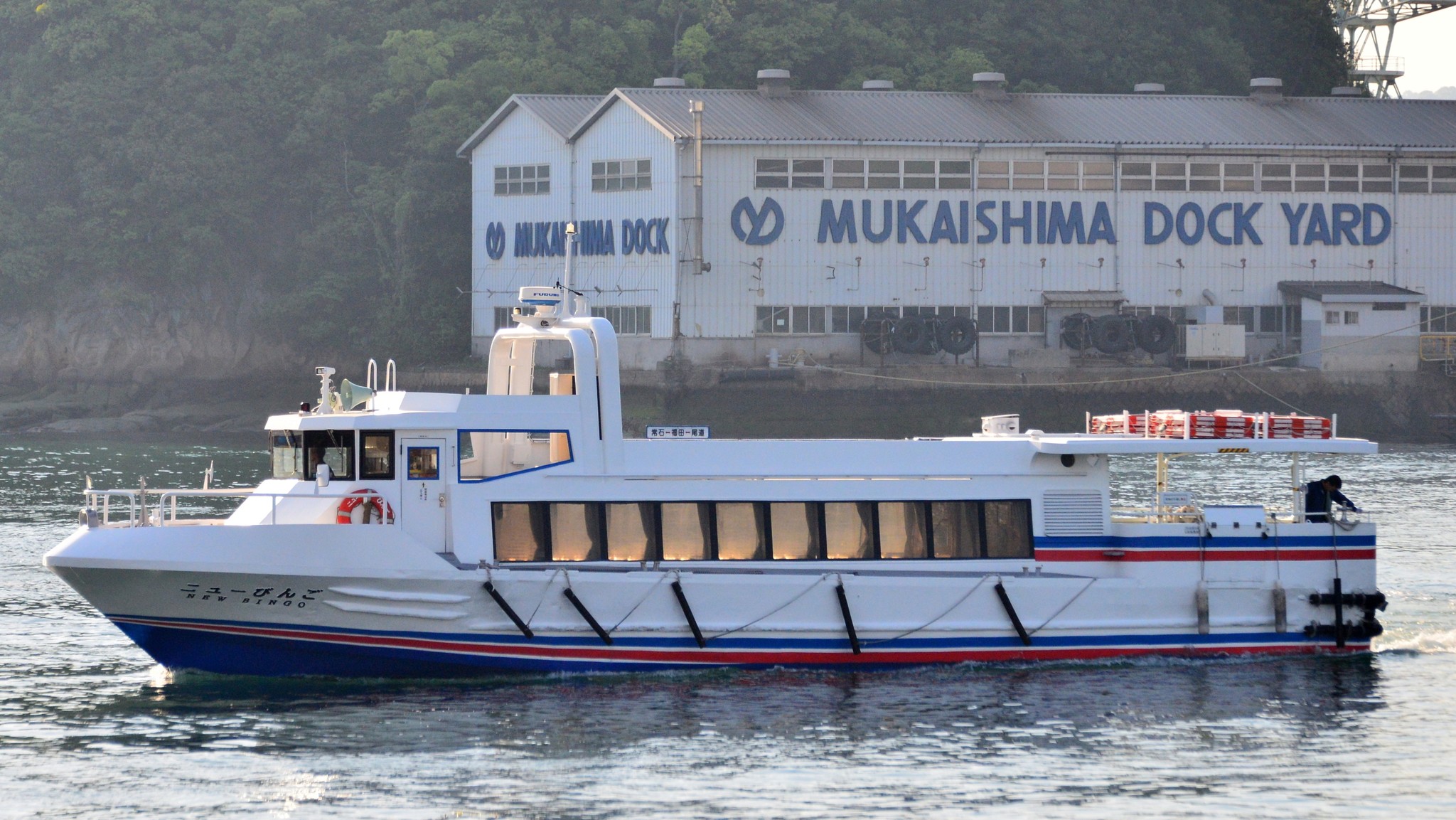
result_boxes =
[340,378,377,412]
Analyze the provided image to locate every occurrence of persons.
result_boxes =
[1305,475,1361,524]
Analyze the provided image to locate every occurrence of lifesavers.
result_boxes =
[917,313,943,355]
[1091,315,1129,353]
[893,315,928,353]
[1119,313,1142,350]
[1062,313,1095,350]
[1135,314,1176,353]
[337,489,394,525]
[940,315,975,355]
[864,312,900,353]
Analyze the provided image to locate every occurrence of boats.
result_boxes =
[42,231,1393,698]
